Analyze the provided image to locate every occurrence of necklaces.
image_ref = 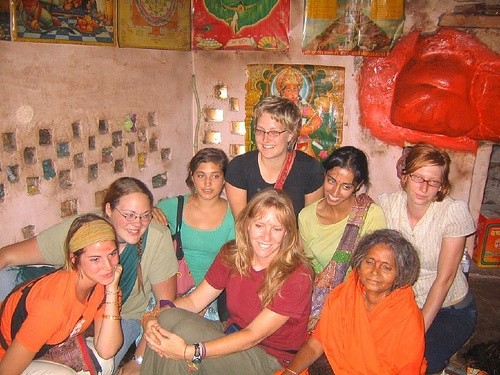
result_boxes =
[117,240,126,244]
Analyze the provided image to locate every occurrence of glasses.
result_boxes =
[409,173,442,188]
[255,128,288,136]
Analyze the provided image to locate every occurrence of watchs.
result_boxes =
[192,343,202,363]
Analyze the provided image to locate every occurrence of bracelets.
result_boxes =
[105,286,120,294]
[141,311,160,327]
[102,314,122,320]
[286,367,298,375]
[184,342,206,373]
[159,299,176,308]
[105,302,119,306]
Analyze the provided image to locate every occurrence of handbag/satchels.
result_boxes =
[308,193,374,336]
[38,333,102,375]
[166,195,196,297]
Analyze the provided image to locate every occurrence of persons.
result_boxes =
[297,146,389,375]
[140,187,315,375]
[0,176,180,374]
[272,229,427,375]
[150,148,236,325]
[0,214,124,375]
[225,95,325,228]
[375,141,477,375]
